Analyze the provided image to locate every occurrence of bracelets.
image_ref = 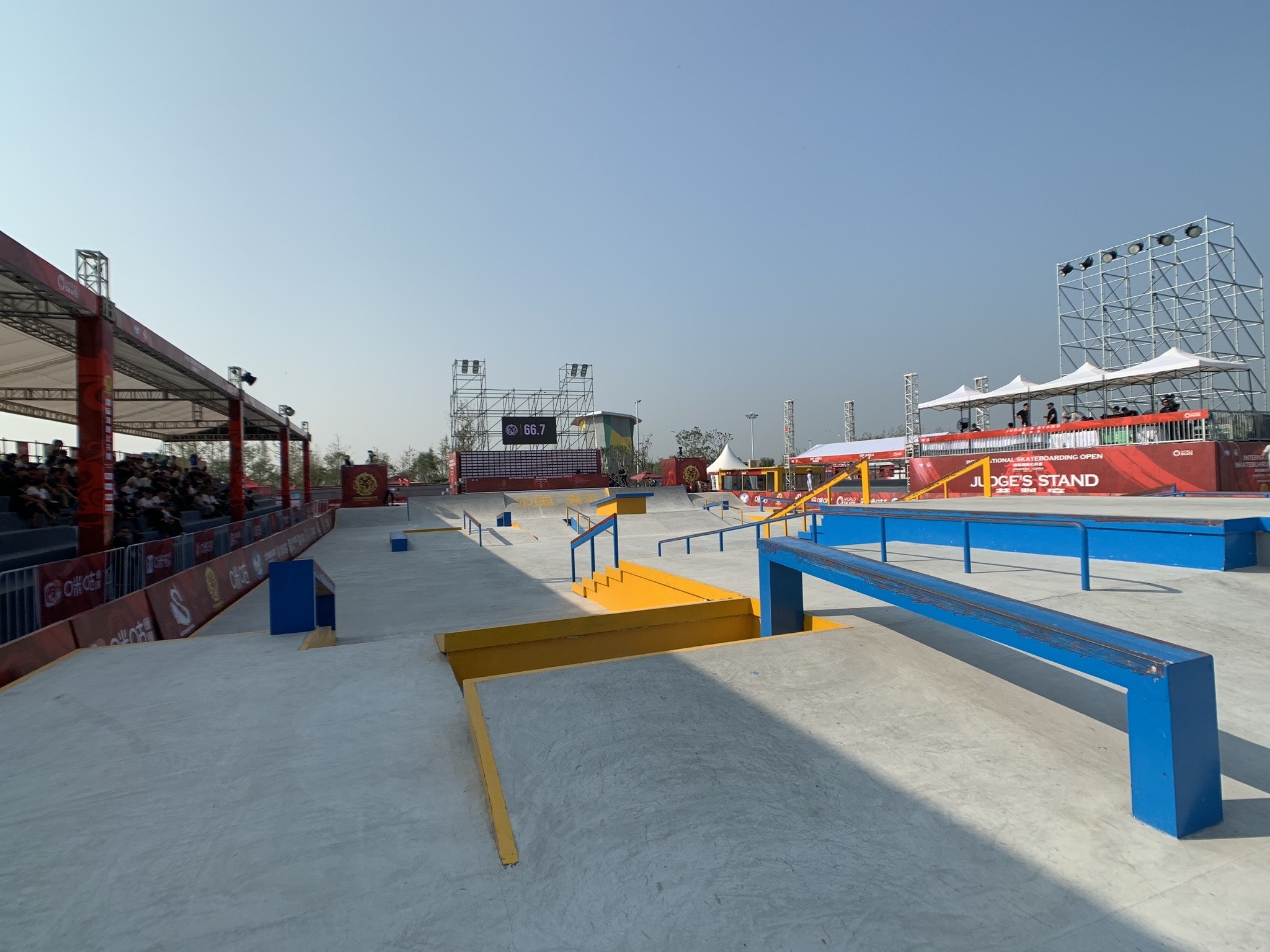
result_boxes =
[1049,420,1051,423]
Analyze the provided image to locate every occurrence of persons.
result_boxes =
[687,480,702,493]
[706,443,748,490]
[1015,403,1031,427]
[806,468,813,491]
[609,466,630,488]
[382,488,400,506]
[1122,407,1145,443]
[648,478,657,487]
[1060,411,1083,432]
[0,439,259,594]
[1160,399,1184,443]
[1043,402,1058,439]
[345,455,354,468]
[1008,423,1015,429]
[970,423,981,433]
[1113,406,1122,415]
[732,479,751,490]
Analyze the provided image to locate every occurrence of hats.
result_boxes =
[806,468,811,471]
[972,423,977,426]
[1047,402,1054,405]
[118,491,132,497]
[1024,403,1030,407]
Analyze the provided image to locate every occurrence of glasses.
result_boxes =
[1164,401,1169,403]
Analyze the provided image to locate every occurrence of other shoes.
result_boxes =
[59,513,71,520]
[50,515,58,521]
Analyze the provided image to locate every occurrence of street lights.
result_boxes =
[745,412,758,460]
[633,399,642,487]
[808,440,812,449]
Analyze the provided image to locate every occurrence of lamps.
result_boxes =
[1059,262,1074,277]
[240,372,256,386]
[571,363,578,377]
[1184,224,1204,239]
[1127,240,1144,255]
[1102,248,1118,263]
[580,363,588,376]
[472,360,479,374]
[1155,231,1175,246]
[1079,256,1093,271]
[286,406,294,416]
[461,359,469,373]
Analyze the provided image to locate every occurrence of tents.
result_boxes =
[918,347,1250,457]
[789,432,956,491]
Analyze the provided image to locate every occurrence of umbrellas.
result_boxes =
[630,471,662,487]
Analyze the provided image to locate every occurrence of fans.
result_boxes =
[957,417,971,433]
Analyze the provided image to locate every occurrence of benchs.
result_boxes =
[0,492,282,640]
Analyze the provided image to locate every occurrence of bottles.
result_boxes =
[1024,422,1026,427]
[1035,422,1038,426]
